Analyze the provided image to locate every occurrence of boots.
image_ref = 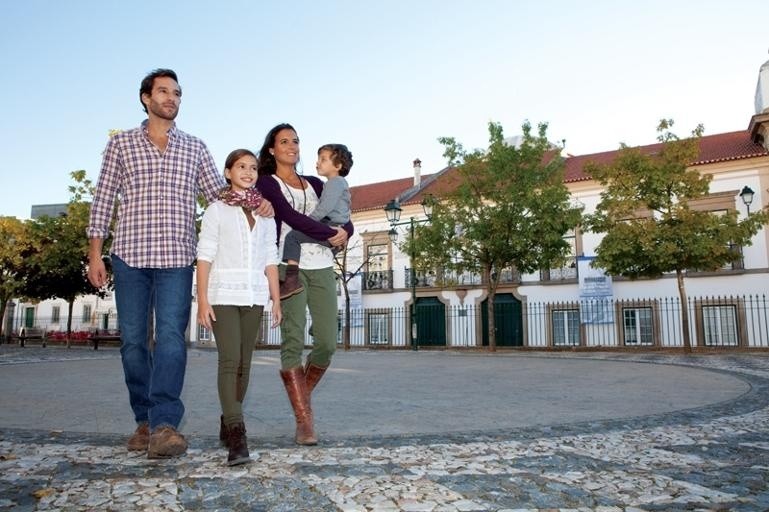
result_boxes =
[279,365,332,446]
[270,265,304,300]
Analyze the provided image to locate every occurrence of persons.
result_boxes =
[88,69,277,460]
[198,148,283,465]
[277,143,353,299]
[255,123,355,444]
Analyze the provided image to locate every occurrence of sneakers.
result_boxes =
[127,423,149,452]
[149,424,189,460]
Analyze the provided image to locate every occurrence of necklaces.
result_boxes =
[275,170,308,215]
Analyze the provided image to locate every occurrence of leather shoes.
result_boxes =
[220,413,252,467]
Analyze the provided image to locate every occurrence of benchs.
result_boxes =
[86,328,121,351]
[18,327,50,348]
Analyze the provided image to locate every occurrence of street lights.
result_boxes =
[384,193,443,350]
[739,185,755,217]
